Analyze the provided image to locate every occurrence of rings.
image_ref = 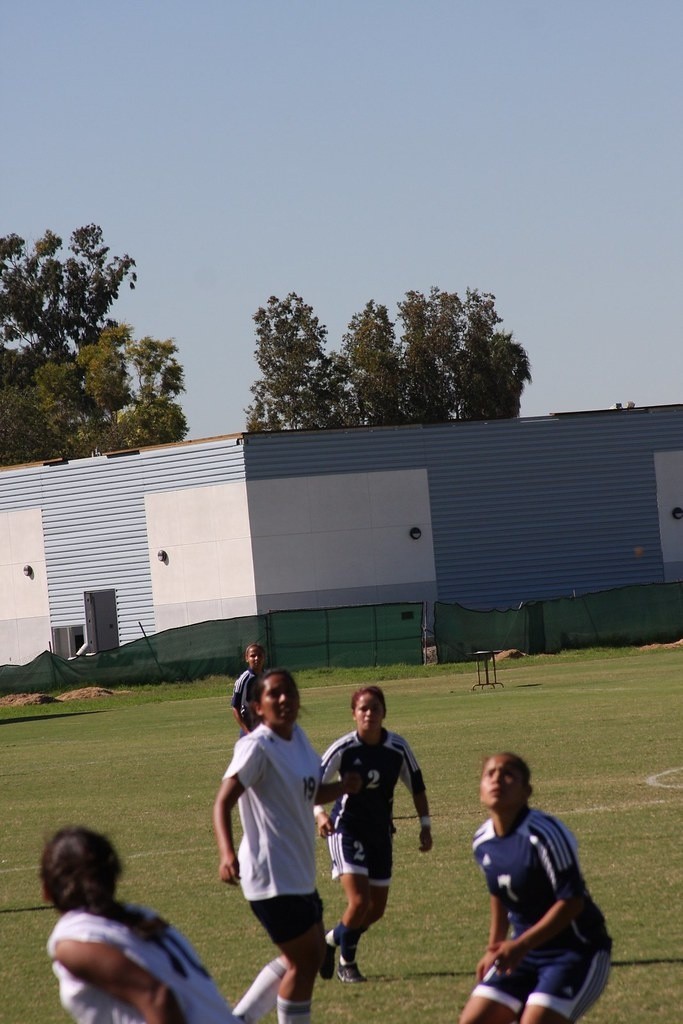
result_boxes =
[494,959,501,966]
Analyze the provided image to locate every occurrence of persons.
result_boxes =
[311,685,433,983]
[41,827,242,1024]
[212,669,362,1024]
[229,644,267,739]
[459,753,613,1024]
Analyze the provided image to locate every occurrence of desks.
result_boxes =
[467,650,505,692]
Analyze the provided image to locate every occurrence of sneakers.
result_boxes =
[337,962,367,982]
[319,930,336,979]
